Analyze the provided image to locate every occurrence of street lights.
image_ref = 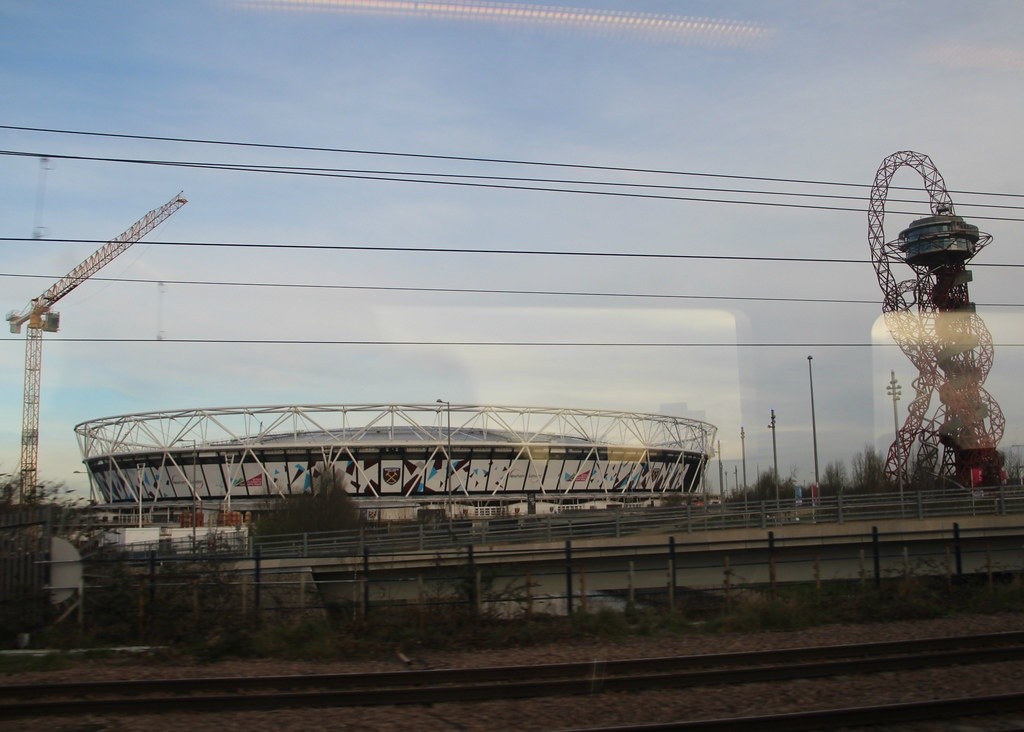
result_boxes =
[435,398,453,539]
[176,438,197,555]
[73,470,94,502]
[806,354,820,485]
[887,370,906,517]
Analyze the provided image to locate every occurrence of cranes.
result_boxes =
[4,187,191,502]
